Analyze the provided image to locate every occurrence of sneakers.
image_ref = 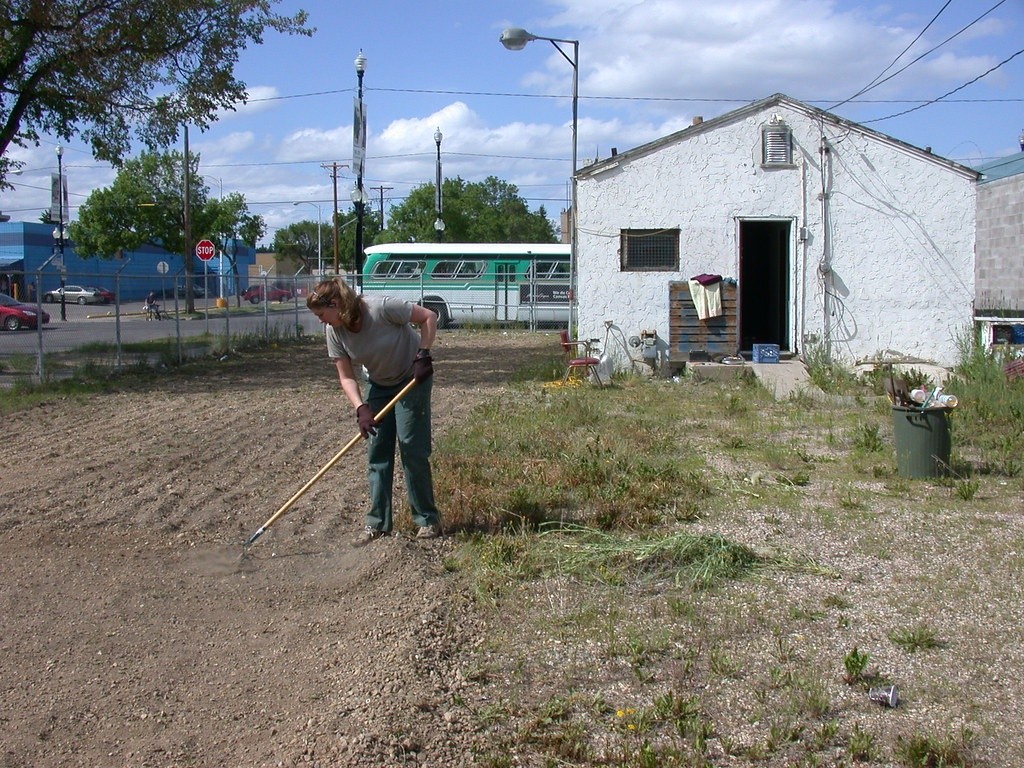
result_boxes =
[416,517,444,539]
[350,526,391,547]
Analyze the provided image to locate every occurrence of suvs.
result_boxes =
[243,283,293,304]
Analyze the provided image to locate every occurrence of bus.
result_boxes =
[350,242,576,330]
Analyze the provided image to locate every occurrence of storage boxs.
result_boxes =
[752,344,780,363]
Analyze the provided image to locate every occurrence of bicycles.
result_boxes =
[144,303,169,322]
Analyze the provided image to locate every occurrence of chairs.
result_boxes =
[559,329,603,388]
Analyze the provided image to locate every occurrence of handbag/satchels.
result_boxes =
[690,273,722,287]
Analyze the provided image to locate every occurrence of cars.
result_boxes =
[0,292,50,331]
[81,285,116,304]
[43,284,100,305]
[177,280,211,299]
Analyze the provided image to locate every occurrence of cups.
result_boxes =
[939,394,958,407]
[912,389,925,401]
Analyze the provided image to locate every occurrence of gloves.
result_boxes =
[357,404,384,439]
[413,356,434,385]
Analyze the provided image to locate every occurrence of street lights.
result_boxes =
[199,173,224,299]
[293,200,322,283]
[432,125,446,242]
[498,26,581,347]
[346,47,370,296]
[52,141,71,321]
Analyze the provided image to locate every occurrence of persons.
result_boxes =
[305,276,443,548]
[144,292,161,321]
[30,282,37,302]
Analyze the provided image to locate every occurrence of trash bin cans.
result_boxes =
[216,298,224,307]
[891,404,955,479]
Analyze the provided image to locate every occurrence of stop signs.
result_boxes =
[195,239,216,261]
